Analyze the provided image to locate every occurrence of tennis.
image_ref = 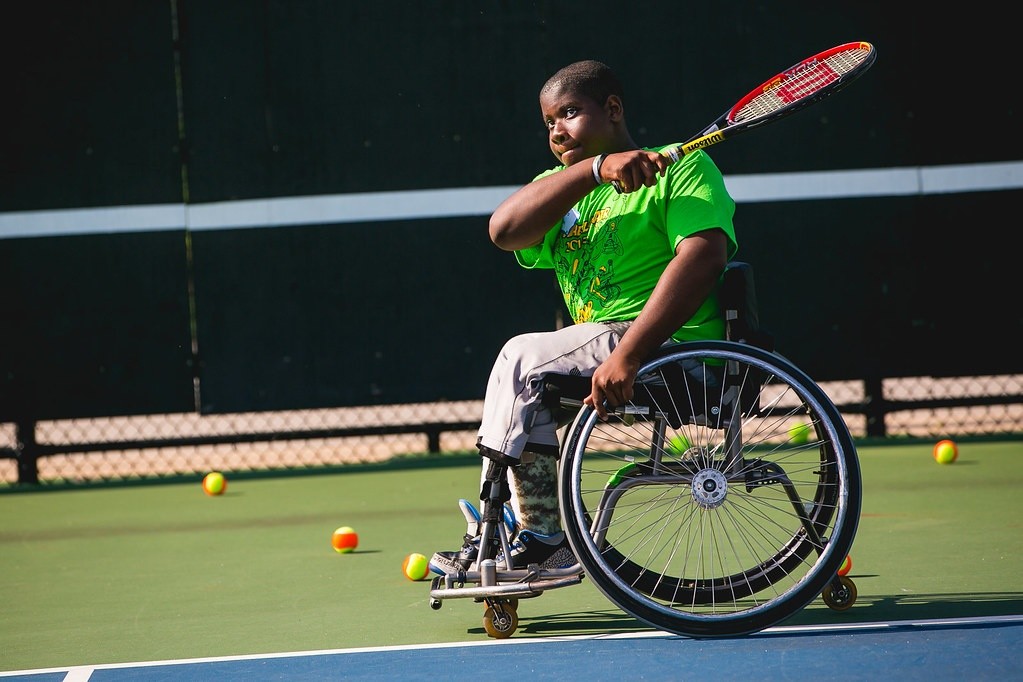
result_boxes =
[202,471,228,497]
[670,435,693,456]
[403,552,430,581]
[836,556,852,578]
[932,439,959,465]
[786,423,810,446]
[331,526,359,553]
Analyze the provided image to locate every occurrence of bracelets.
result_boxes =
[592,152,611,184]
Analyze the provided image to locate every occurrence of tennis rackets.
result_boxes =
[610,37,877,196]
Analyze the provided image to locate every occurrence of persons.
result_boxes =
[429,58,739,578]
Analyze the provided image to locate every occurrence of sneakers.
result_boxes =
[428,498,484,576]
[469,502,580,574]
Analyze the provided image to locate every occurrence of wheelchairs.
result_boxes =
[427,260,862,639]
